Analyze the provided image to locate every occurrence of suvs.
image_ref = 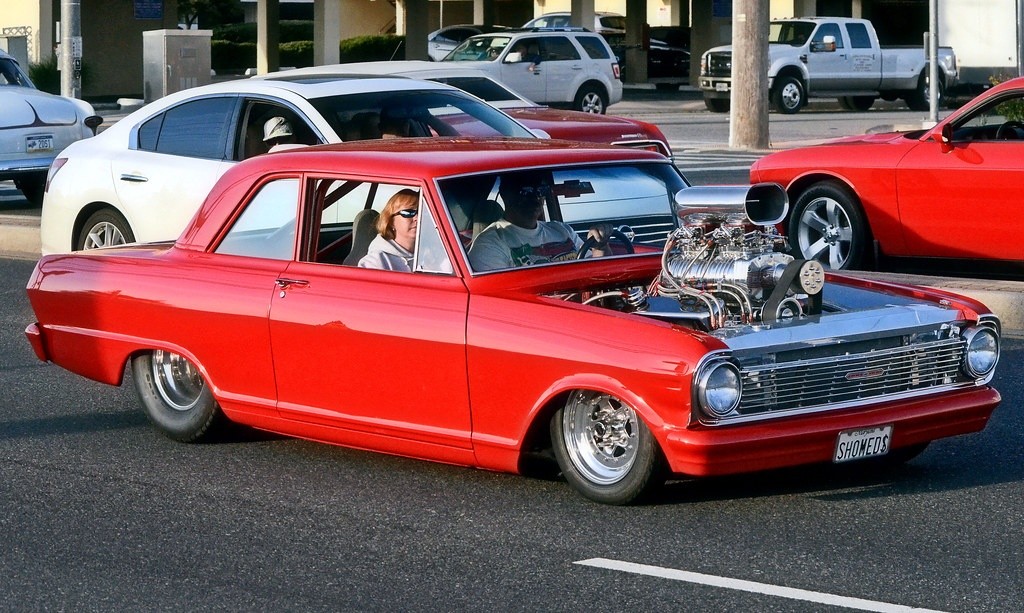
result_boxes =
[442,28,622,115]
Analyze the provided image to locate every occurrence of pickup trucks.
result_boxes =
[697,16,958,111]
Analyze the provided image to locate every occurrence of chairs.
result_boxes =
[342,208,379,265]
[527,43,541,62]
[471,200,505,244]
[340,112,382,143]
[245,124,267,161]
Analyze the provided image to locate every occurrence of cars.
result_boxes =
[0,49,102,203]
[249,60,673,157]
[600,32,690,91]
[39,72,676,258]
[521,11,626,34]
[747,77,1024,272]
[427,24,514,62]
[27,138,1004,506]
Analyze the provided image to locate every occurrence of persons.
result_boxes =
[377,104,410,139]
[262,117,293,151]
[516,42,541,72]
[358,189,419,273]
[467,170,613,272]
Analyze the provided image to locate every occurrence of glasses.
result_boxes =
[504,184,550,198]
[391,207,419,218]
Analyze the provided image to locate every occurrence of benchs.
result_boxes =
[215,225,353,265]
